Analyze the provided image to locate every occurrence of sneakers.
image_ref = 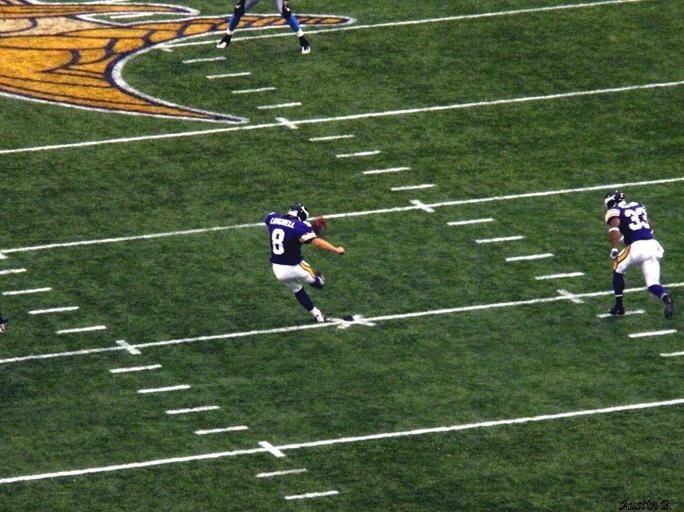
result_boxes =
[608,306,624,315]
[298,34,311,54]
[664,295,673,320]
[316,312,327,323]
[216,34,231,48]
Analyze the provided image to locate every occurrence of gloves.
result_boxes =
[235,3,244,16]
[282,1,291,19]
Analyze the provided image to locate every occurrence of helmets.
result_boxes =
[287,203,309,221]
[605,191,625,209]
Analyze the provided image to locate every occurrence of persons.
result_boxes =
[215,0,310,56]
[601,189,676,323]
[264,202,344,323]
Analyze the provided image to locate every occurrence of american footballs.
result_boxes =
[312,219,326,231]
